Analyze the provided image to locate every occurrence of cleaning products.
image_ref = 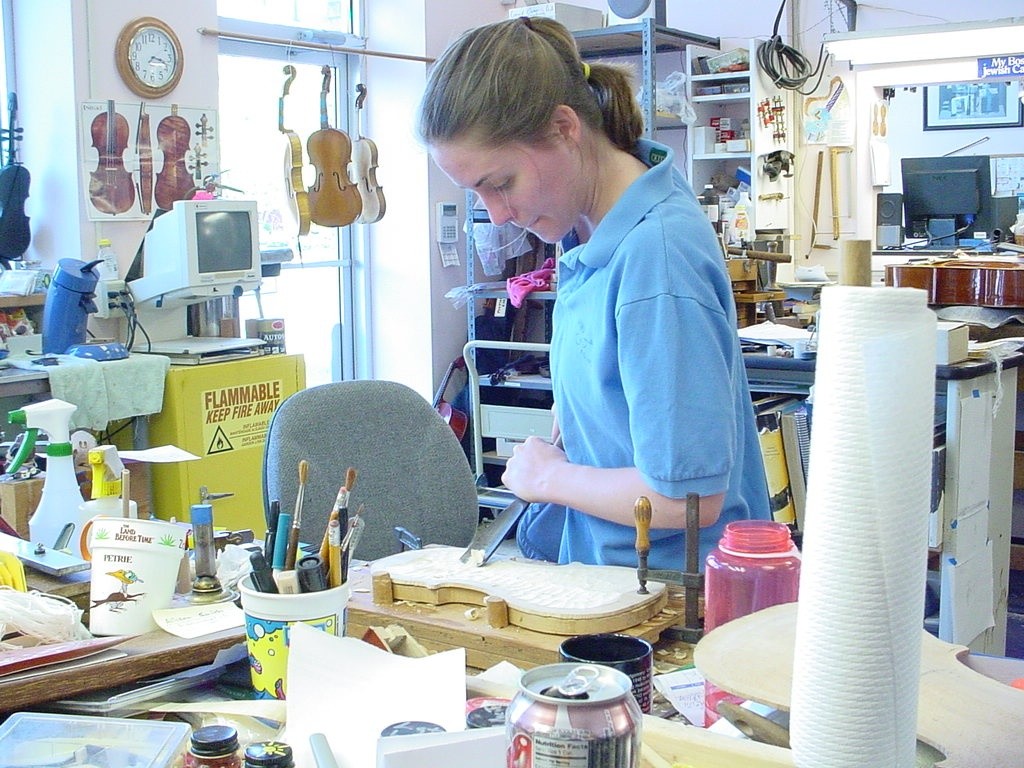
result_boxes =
[5,398,85,559]
[75,445,138,562]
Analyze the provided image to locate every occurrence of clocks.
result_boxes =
[116,16,184,98]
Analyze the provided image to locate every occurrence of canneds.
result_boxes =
[505,662,643,768]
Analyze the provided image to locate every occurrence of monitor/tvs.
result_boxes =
[902,155,992,250]
[144,199,261,293]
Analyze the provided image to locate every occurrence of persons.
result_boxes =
[420,16,774,571]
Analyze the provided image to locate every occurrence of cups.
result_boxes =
[559,633,654,715]
[238,574,350,699]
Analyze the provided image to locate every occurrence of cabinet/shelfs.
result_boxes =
[467,19,720,508]
[91,354,306,537]
[684,38,788,283]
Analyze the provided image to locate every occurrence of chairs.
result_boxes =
[263,380,478,561]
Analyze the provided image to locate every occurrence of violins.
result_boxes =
[348,84,385,224]
[269,64,310,237]
[0,90,32,264]
[306,65,362,228]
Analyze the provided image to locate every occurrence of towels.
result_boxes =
[8,350,172,432]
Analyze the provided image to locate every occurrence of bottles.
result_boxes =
[702,519,801,729]
[189,725,242,768]
[96,240,118,282]
[693,183,756,248]
[19,259,44,292]
[244,741,295,768]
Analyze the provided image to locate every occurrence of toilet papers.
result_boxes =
[788,284,938,768]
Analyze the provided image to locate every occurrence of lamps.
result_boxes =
[822,16,1024,66]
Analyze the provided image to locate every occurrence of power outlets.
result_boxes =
[95,281,126,319]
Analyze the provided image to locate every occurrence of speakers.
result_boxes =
[876,193,903,247]
[988,195,1019,244]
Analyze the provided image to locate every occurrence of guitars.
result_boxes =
[885,258,1024,309]
[431,356,469,443]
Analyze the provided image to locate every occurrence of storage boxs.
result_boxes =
[496,438,524,457]
[0,463,145,543]
[481,404,552,438]
[936,322,968,365]
[696,117,751,154]
[0,711,192,768]
[719,164,752,248]
[509,3,603,31]
[693,48,750,94]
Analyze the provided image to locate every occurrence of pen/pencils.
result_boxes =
[249,459,357,594]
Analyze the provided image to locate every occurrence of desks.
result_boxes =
[0,366,151,514]
[745,352,1024,658]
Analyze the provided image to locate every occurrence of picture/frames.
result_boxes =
[923,80,1024,131]
